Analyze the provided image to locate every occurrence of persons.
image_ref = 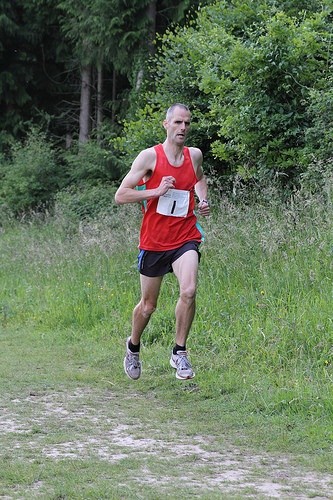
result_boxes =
[115,103,211,380]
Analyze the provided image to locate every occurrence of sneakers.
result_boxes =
[123,336,142,380]
[169,348,195,380]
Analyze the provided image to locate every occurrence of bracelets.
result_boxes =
[201,200,208,203]
